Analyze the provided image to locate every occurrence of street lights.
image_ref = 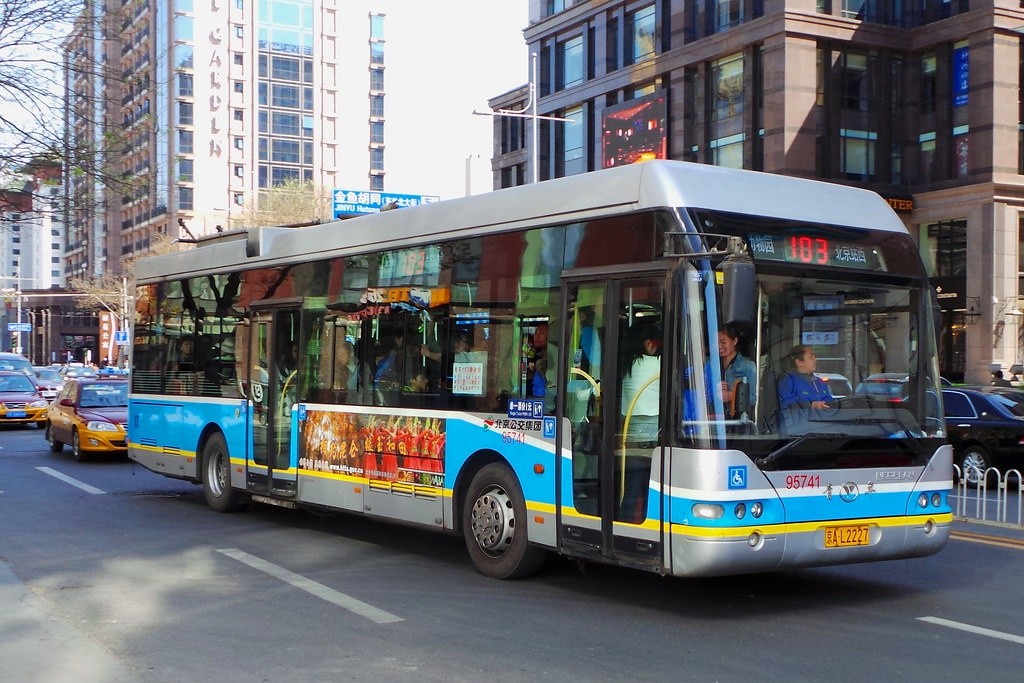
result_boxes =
[473,52,576,184]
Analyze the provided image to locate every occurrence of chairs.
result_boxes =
[762,368,780,421]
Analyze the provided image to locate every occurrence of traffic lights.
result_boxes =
[11,336,17,348]
[83,348,87,356]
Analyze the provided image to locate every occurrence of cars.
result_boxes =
[0,352,129,429]
[901,384,1024,490]
[812,370,852,399]
[45,367,132,463]
[854,372,953,403]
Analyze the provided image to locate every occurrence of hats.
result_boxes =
[215,337,237,354]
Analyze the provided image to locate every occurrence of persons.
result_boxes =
[147,329,235,371]
[349,414,446,487]
[533,323,548,399]
[615,316,758,522]
[777,345,835,411]
[281,340,297,416]
[334,325,469,393]
[992,370,1012,388]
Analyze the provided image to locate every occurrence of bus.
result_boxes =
[126,157,955,582]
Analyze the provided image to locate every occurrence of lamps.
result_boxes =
[965,296,982,325]
[1009,365,1024,376]
[1005,295,1024,324]
